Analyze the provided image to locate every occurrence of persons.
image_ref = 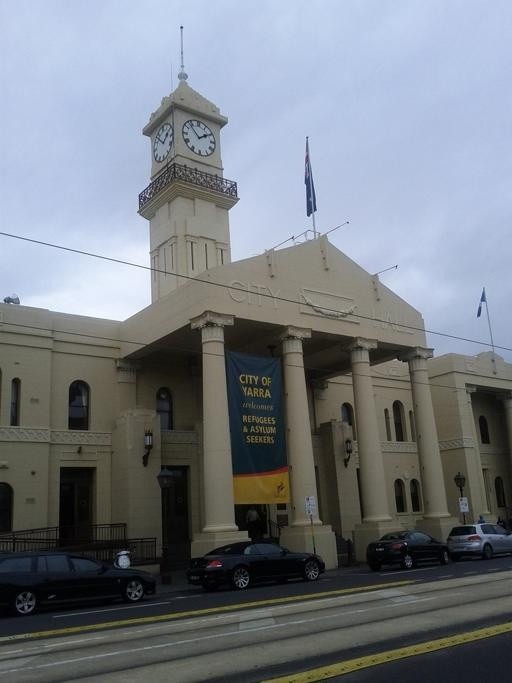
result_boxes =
[476,514,485,524]
[246,504,261,536]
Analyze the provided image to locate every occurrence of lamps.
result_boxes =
[343,437,353,468]
[142,428,154,466]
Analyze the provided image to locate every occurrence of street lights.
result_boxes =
[454,471,467,525]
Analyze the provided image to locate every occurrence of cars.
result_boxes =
[1,551,157,615]
[365,530,450,571]
[186,540,326,590]
[446,522,512,559]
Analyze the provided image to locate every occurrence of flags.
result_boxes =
[304,138,317,216]
[476,288,485,318]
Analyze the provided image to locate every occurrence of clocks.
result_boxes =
[181,118,216,157]
[153,121,174,163]
